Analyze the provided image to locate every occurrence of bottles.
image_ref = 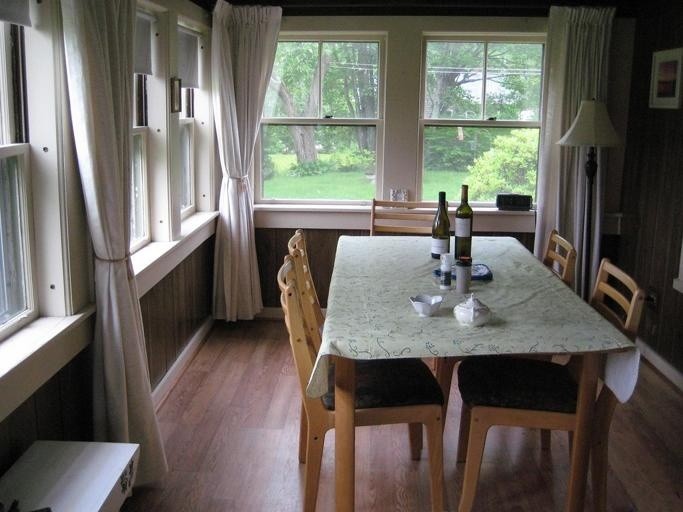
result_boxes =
[454,184,474,261]
[439,253,453,290]
[430,191,451,260]
[455,261,472,292]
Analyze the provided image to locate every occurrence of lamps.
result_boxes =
[554,99,624,305]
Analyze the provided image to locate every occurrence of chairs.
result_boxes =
[458,258,643,511]
[287,229,331,359]
[369,198,448,237]
[418,230,577,431]
[278,256,444,512]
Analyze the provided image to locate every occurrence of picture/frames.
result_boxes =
[648,48,681,111]
[390,189,409,210]
[171,78,183,113]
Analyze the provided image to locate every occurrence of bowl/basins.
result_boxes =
[409,294,442,317]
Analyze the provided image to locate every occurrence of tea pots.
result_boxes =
[453,293,492,328]
